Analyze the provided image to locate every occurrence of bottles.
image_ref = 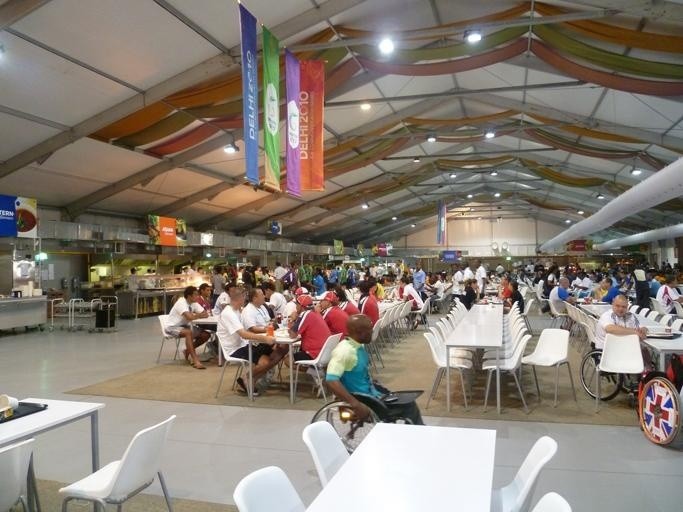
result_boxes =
[266,323,273,337]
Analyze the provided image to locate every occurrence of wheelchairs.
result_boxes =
[310,366,427,456]
[579,329,664,405]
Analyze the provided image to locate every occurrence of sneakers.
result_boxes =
[183,350,189,364]
[193,364,205,369]
[252,388,258,395]
[236,377,246,393]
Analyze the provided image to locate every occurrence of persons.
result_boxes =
[127,257,683,397]
[324,313,425,425]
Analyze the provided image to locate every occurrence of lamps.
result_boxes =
[462,28,482,44]
[483,128,496,139]
[222,135,240,154]
[426,134,436,143]
[490,169,498,176]
[361,202,370,209]
[413,157,420,163]
[629,165,642,176]
[596,192,604,200]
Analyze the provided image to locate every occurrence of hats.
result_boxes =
[315,291,338,301]
[292,295,313,308]
[294,287,309,295]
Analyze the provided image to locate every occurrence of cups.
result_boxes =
[664,327,671,335]
[0,395,19,411]
